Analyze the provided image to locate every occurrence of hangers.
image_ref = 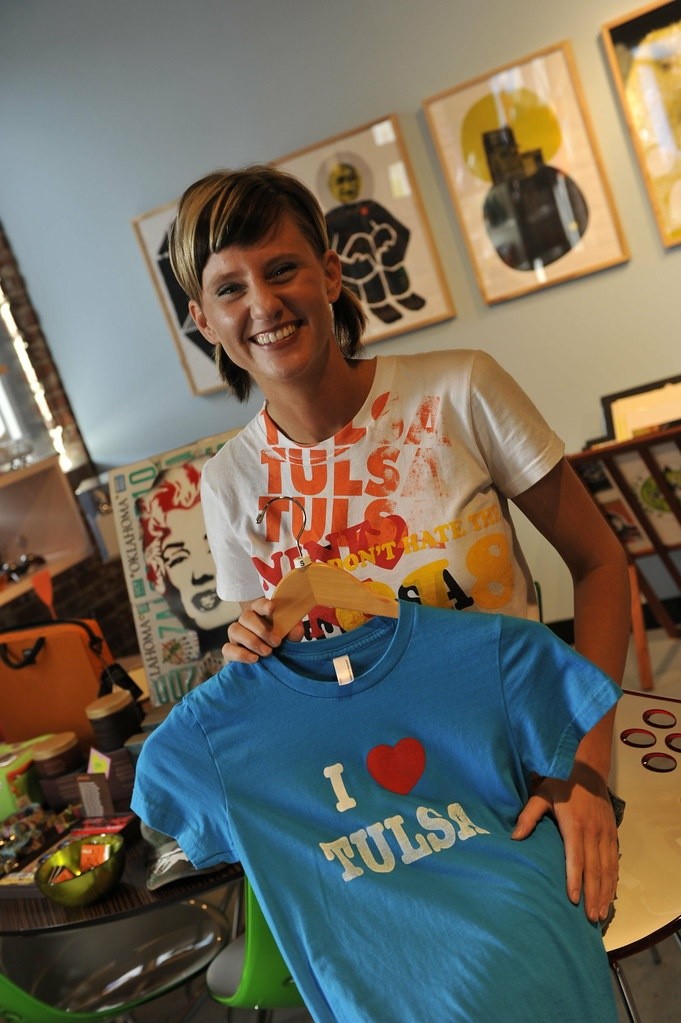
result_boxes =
[252,488,400,654]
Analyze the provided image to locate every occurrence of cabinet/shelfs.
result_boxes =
[0,451,90,607]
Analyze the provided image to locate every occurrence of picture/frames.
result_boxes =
[422,40,629,305]
[599,0,681,249]
[265,113,457,350]
[132,197,254,397]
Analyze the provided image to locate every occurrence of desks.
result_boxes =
[574,686,681,1023]
[0,826,274,1023]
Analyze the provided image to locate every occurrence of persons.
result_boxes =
[165,165,631,923]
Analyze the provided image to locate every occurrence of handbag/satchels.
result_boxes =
[0,618,144,744]
